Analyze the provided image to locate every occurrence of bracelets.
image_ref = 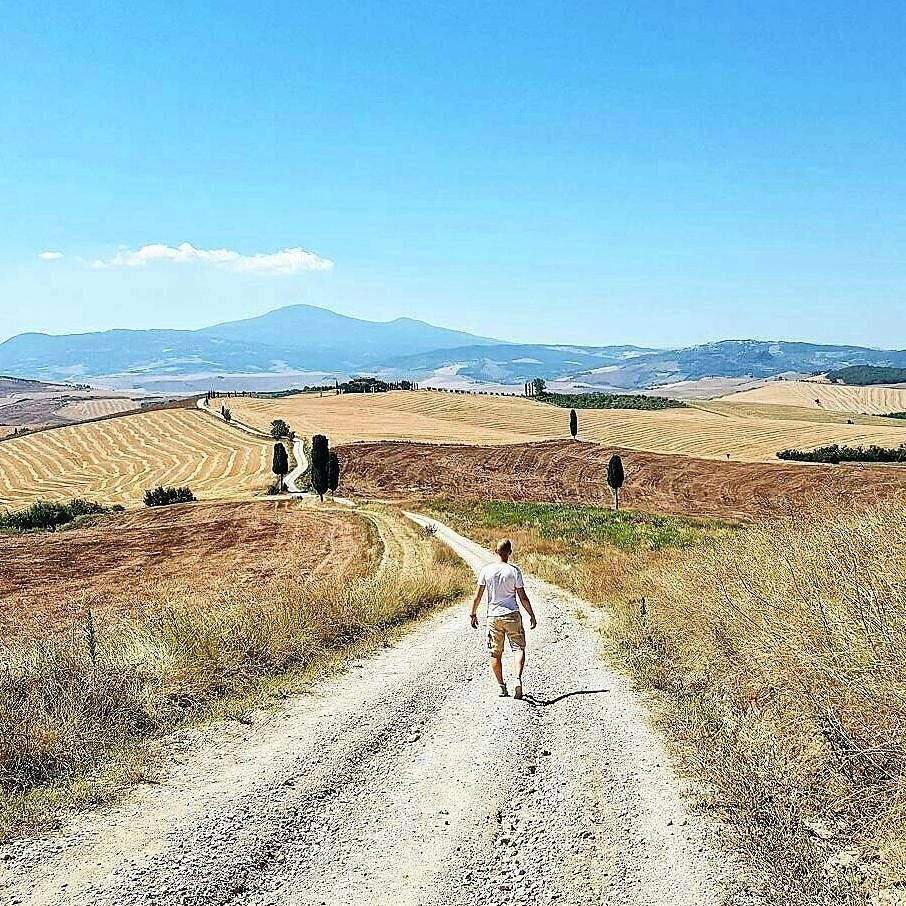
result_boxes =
[470,614,476,617]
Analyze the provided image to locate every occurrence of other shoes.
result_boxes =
[514,678,523,699]
[499,683,509,697]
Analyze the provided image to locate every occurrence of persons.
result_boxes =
[471,538,536,699]
